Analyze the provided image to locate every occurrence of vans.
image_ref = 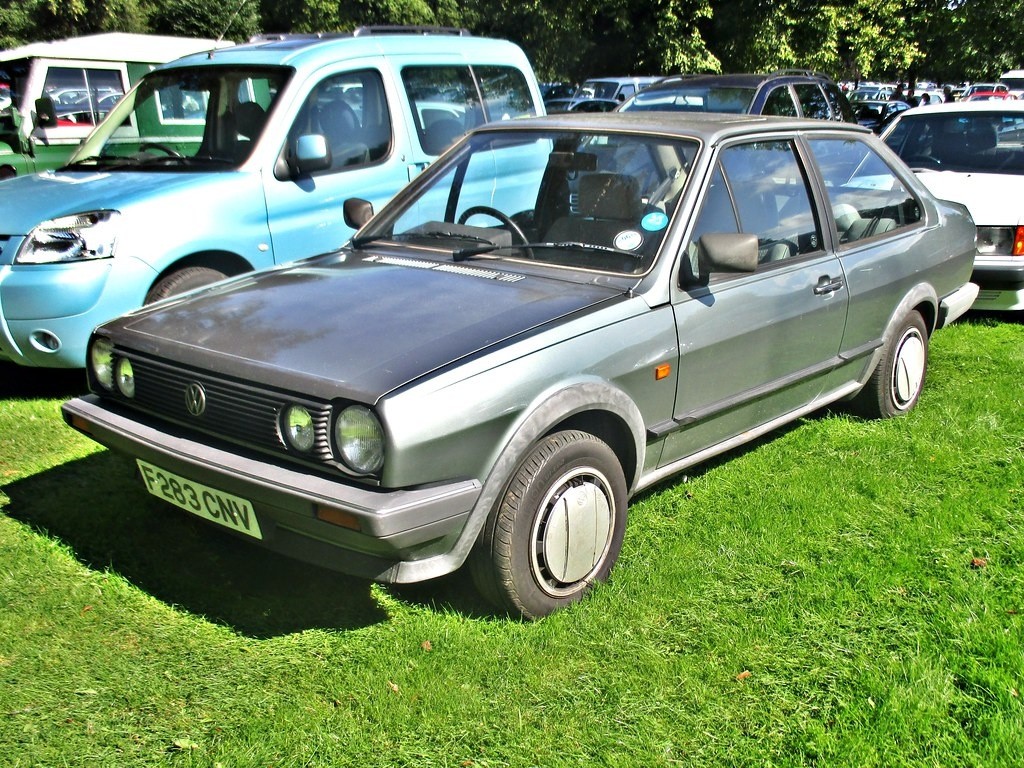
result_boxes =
[0,31,271,183]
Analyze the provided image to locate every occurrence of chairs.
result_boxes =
[317,101,370,167]
[233,102,265,163]
[927,134,966,170]
[535,171,647,274]
[690,176,795,277]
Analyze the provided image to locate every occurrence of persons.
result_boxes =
[889,85,956,109]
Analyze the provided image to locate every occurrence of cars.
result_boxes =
[60,110,985,618]
[0,27,555,373]
[416,68,1024,150]
[831,99,1024,313]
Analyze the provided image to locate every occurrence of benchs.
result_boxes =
[640,164,897,243]
[359,119,465,156]
[974,148,1024,169]
